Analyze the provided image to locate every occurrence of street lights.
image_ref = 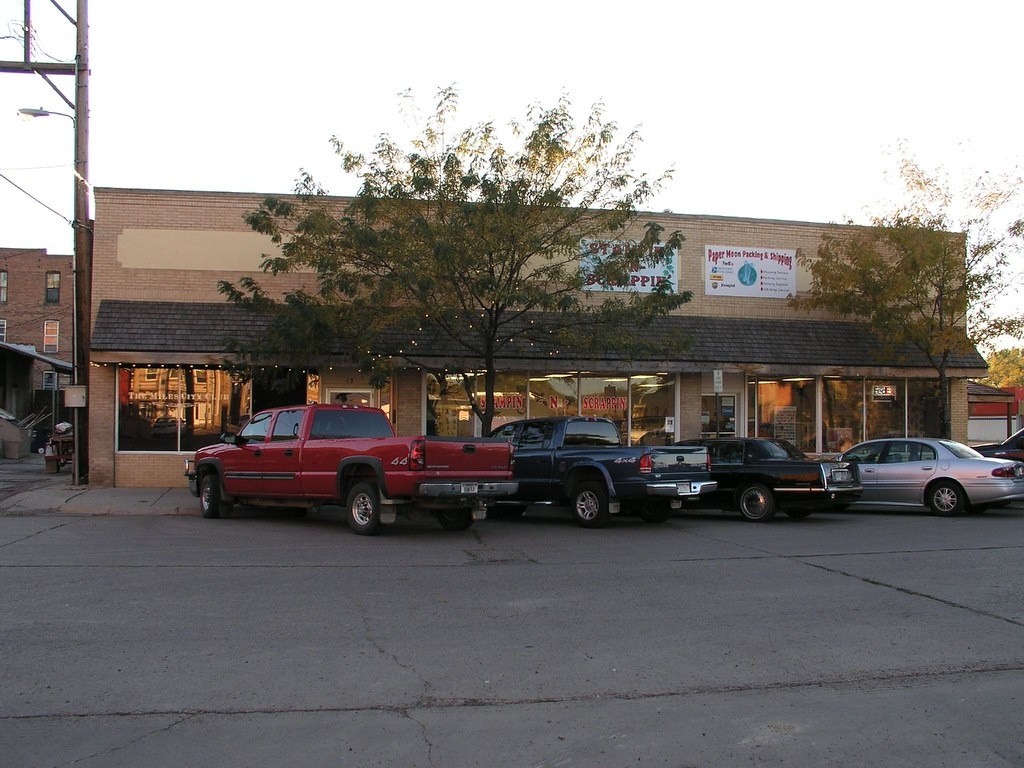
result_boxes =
[17,107,90,484]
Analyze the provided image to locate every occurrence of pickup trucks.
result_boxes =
[480,416,719,530]
[183,402,519,537]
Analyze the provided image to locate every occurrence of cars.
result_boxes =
[668,436,864,523]
[153,416,187,438]
[969,429,1024,461]
[829,437,1023,517]
